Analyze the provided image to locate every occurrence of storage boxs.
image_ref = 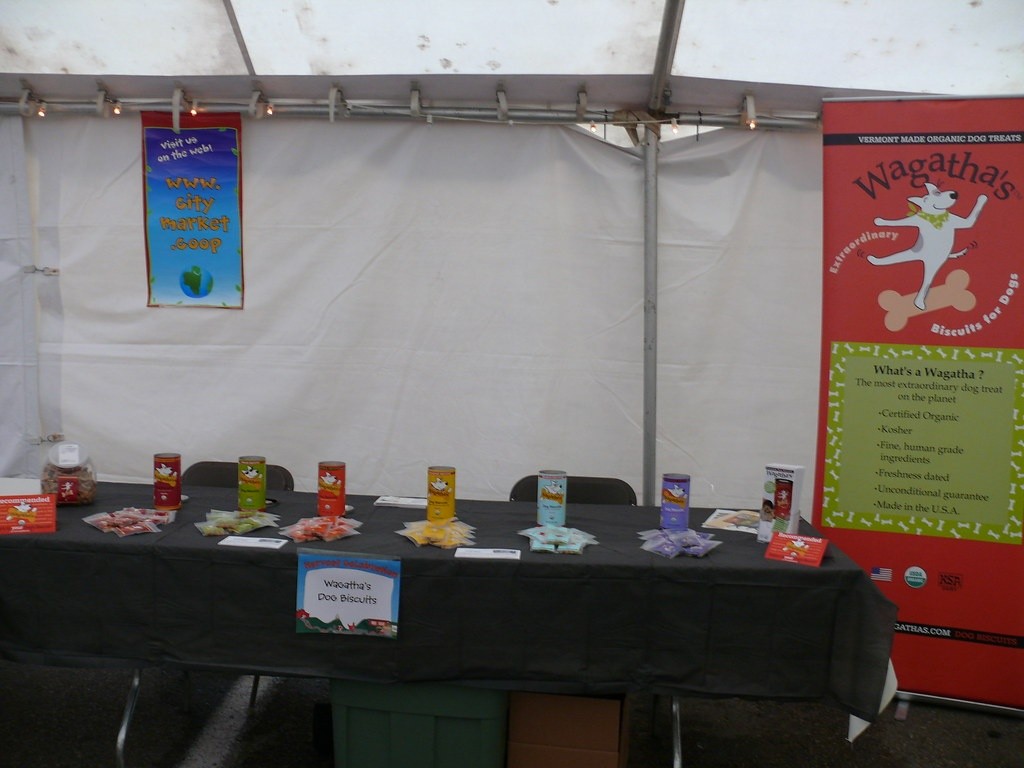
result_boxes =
[507,687,625,768]
[327,678,506,768]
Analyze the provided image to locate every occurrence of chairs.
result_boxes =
[180,461,294,712]
[508,473,637,507]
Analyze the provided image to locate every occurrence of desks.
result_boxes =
[0,480,862,768]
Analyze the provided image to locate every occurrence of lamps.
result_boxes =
[190,99,199,118]
[744,96,757,130]
[37,101,47,117]
[264,104,276,119]
[114,100,122,114]
